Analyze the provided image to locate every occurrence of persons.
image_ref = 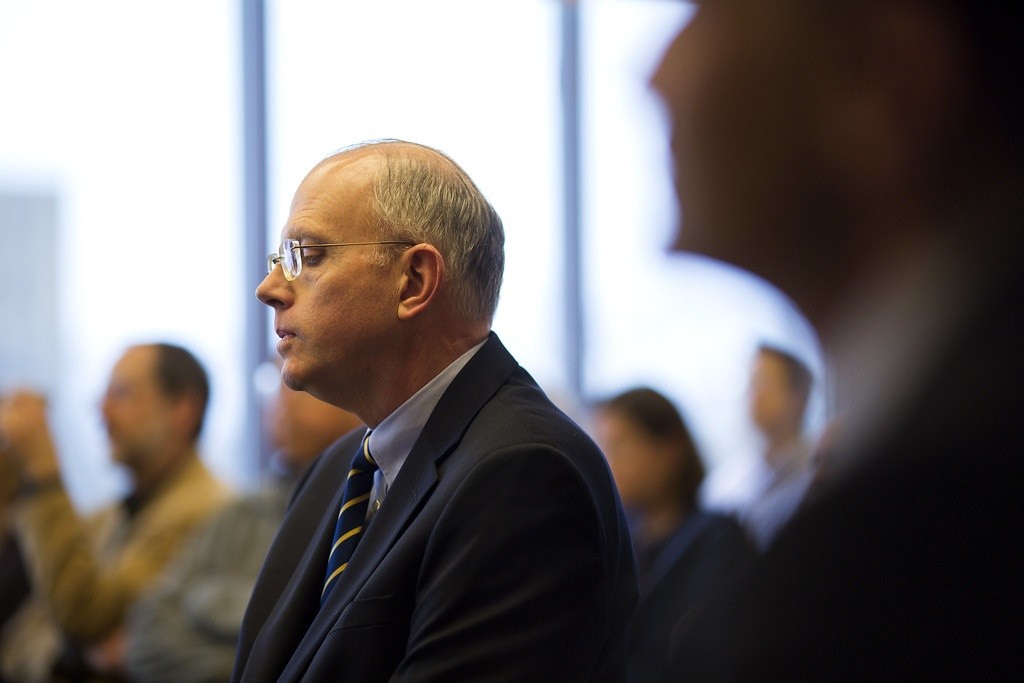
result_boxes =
[589,339,820,682]
[0,339,370,682]
[646,1,1024,683]
[229,139,644,683]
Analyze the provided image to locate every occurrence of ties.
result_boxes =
[322,432,377,612]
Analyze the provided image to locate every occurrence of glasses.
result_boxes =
[266,238,422,281]
